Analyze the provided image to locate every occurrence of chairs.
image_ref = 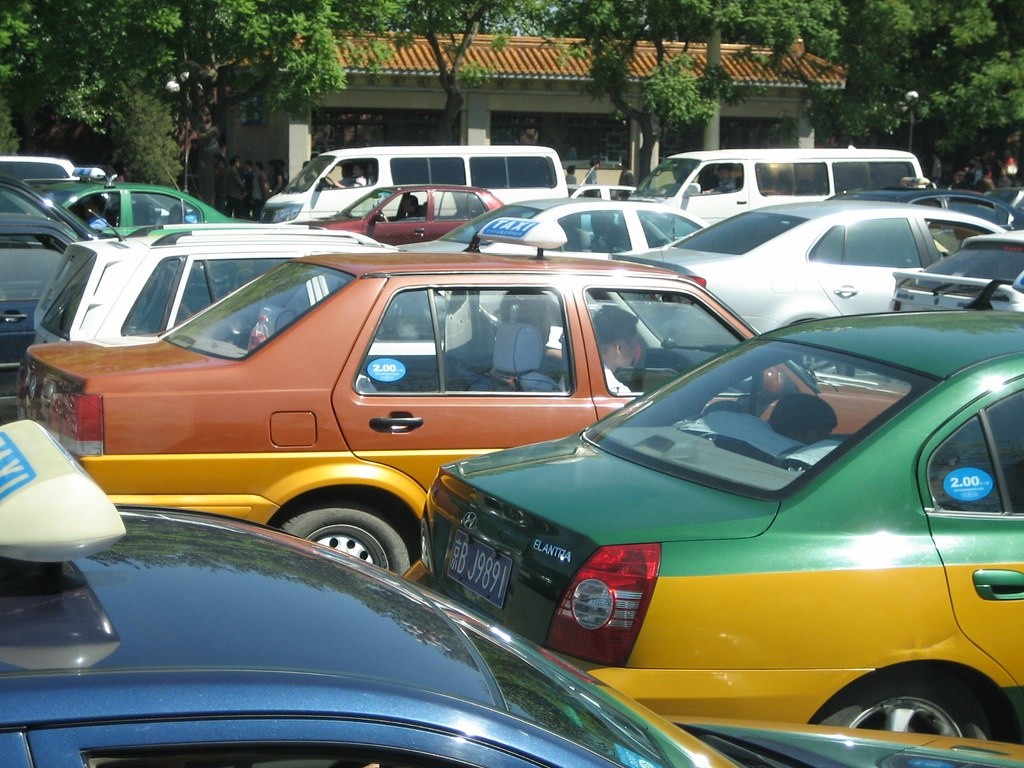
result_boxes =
[570,227,592,253]
[489,321,560,392]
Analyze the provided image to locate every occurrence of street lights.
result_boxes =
[166,70,204,194]
[896,91,921,153]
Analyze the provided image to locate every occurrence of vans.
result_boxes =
[258,145,571,224]
[615,145,926,225]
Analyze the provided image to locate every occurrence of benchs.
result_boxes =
[702,408,843,465]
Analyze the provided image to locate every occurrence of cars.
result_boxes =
[1,420,1024,768]
[404,308,1024,752]
[14,213,909,577]
[398,197,711,262]
[565,183,638,233]
[594,185,1024,380]
[287,183,509,246]
[1,154,394,425]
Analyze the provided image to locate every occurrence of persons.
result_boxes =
[170,205,187,223]
[558,305,638,393]
[566,166,577,195]
[133,202,150,225]
[929,443,962,510]
[949,149,1018,193]
[215,144,289,221]
[702,166,736,194]
[403,195,423,218]
[86,196,108,228]
[618,160,636,198]
[332,162,372,188]
[302,160,317,181]
[585,159,600,197]
[770,391,835,443]
[522,126,537,144]
[920,152,942,186]
[503,290,565,378]
